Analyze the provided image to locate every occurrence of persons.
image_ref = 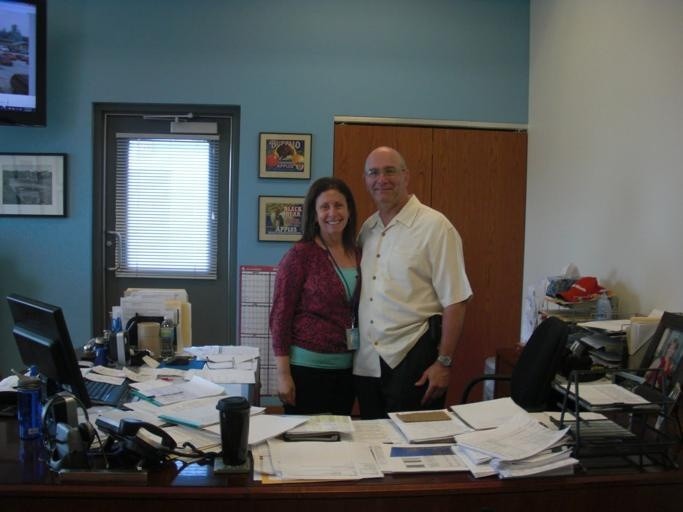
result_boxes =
[644,338,678,390]
[269,177,362,415]
[351,146,473,420]
[266,206,283,233]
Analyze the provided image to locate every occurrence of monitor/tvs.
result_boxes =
[6,292,92,408]
[0,0,47,126]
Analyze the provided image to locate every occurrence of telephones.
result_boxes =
[95,418,177,471]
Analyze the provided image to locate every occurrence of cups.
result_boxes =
[215,395,251,465]
[16,376,43,441]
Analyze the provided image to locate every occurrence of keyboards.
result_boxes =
[85,379,129,407]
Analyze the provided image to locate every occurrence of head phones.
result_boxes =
[39,393,95,461]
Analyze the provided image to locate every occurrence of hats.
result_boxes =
[555,276,607,304]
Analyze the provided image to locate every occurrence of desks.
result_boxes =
[75,348,262,407]
[0,414,667,512]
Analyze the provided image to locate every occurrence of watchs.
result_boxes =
[437,353,453,369]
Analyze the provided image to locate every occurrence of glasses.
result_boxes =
[365,167,404,176]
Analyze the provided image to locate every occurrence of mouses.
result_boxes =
[167,357,189,365]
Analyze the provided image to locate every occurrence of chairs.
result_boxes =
[461,315,569,414]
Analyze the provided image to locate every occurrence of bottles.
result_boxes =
[161,316,175,356]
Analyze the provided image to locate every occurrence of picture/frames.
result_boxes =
[636,312,682,462]
[258,131,312,178]
[1,153,68,218]
[257,196,306,242]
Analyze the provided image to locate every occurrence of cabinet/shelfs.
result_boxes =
[335,121,528,404]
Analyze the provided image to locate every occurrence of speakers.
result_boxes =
[41,390,79,429]
[111,330,130,365]
